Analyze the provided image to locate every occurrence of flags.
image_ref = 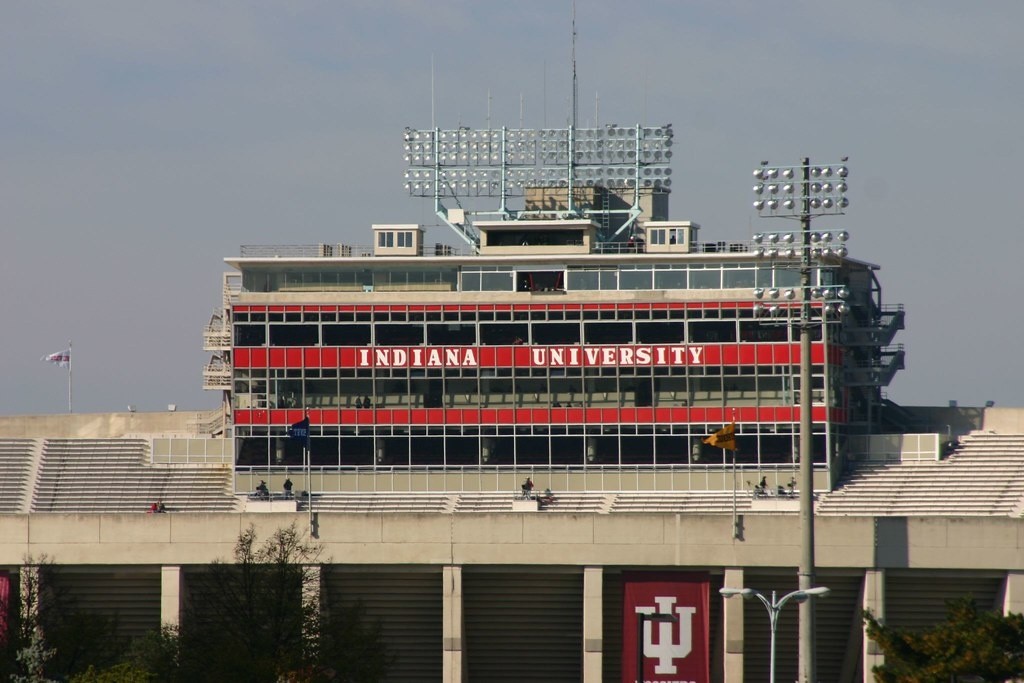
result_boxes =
[38,349,70,370]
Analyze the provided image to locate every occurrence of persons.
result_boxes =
[521,477,534,501]
[355,393,370,408]
[283,478,293,500]
[254,480,270,501]
[153,498,165,512]
[752,475,767,499]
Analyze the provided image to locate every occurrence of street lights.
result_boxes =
[718,587,831,683]
[751,156,850,683]
